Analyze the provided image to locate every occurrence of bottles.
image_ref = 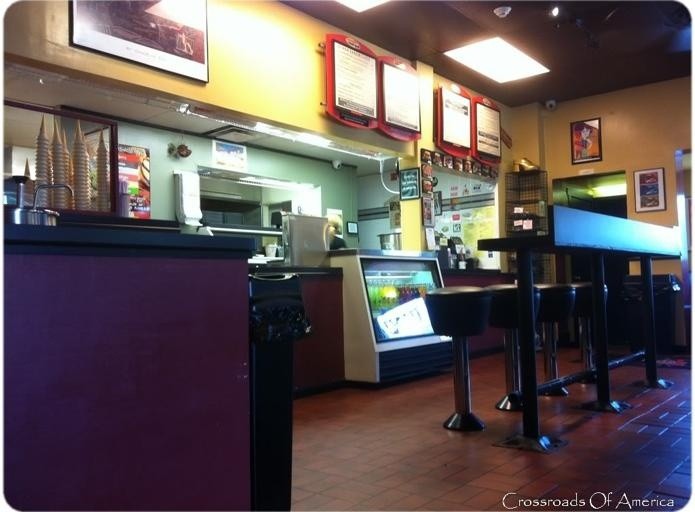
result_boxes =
[463,184,468,196]
[367,280,434,312]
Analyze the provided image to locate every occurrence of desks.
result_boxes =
[475,204,684,455]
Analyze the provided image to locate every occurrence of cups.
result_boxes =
[264,244,277,257]
[458,261,467,269]
[119,192,131,218]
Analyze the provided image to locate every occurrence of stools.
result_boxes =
[422,281,611,434]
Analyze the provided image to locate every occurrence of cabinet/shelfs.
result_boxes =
[503,167,549,237]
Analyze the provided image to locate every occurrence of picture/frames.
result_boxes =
[434,190,443,218]
[419,196,437,228]
[68,0,211,85]
[399,165,422,202]
[569,116,603,165]
[633,167,667,214]
